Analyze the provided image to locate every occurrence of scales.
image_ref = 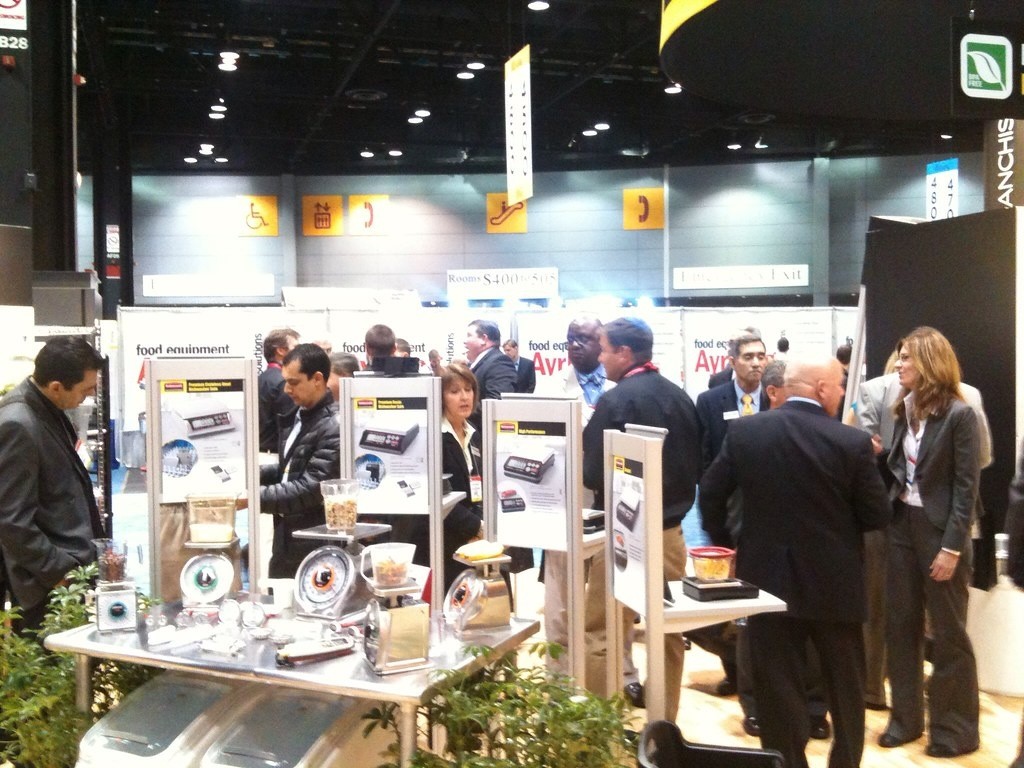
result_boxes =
[175,537,241,632]
[362,578,437,677]
[581,509,605,534]
[503,445,555,484]
[95,576,138,635]
[616,486,640,532]
[358,422,420,455]
[442,554,514,639]
[283,522,393,635]
[440,473,455,499]
[183,409,235,438]
[680,576,760,603]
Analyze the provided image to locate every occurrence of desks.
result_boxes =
[43,590,540,768]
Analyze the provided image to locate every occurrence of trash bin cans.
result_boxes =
[635,720,784,768]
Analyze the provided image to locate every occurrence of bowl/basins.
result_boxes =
[688,545,736,583]
[187,494,239,543]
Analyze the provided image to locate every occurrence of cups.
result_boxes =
[89,538,127,583]
[320,477,360,531]
[361,542,417,587]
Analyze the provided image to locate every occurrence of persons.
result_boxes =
[235,343,342,580]
[0,334,105,667]
[693,330,830,739]
[535,314,707,746]
[258,327,536,599]
[836,326,992,756]
[699,353,893,768]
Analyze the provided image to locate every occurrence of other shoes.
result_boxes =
[865,701,887,710]
[625,682,646,709]
[927,742,961,758]
[880,733,903,748]
[810,719,830,740]
[717,679,737,696]
[743,715,762,736]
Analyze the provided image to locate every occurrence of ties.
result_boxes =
[742,394,752,417]
